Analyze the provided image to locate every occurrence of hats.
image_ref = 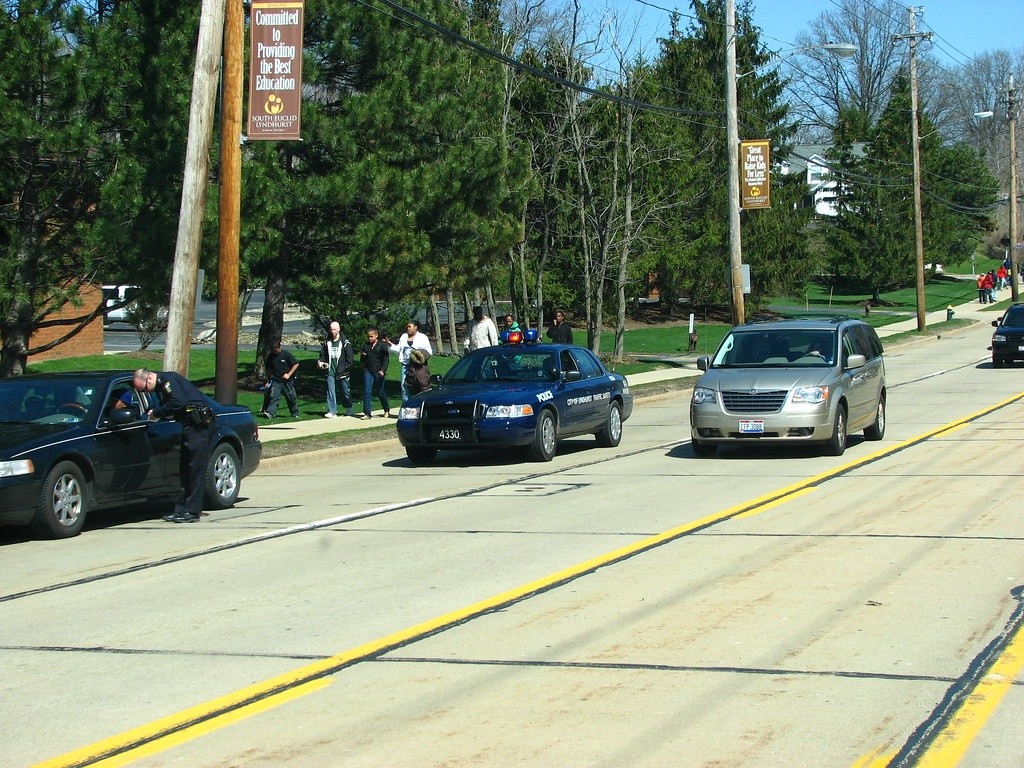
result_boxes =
[981,273,985,275]
[474,307,482,320]
[270,341,281,350]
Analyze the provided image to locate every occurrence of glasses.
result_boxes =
[142,380,147,392]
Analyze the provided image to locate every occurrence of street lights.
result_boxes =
[910,57,993,332]
[726,0,858,326]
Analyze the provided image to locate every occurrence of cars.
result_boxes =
[0,370,262,540]
[395,330,633,465]
[992,303,1024,368]
[924,263,943,278]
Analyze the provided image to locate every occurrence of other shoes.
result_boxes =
[361,415,371,420]
[324,412,337,419]
[384,412,389,419]
[990,301,994,303]
[294,414,299,420]
[979,300,982,303]
[263,412,272,420]
[984,301,986,304]
[346,407,353,416]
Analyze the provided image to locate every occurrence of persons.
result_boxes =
[805,337,831,361]
[316,321,354,418]
[360,328,391,420]
[546,311,573,345]
[100,385,141,426]
[262,341,301,420]
[382,320,432,405]
[977,252,1024,304]
[133,368,215,523]
[501,312,526,345]
[463,306,498,355]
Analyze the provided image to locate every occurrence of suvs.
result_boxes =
[103,285,169,332]
[690,313,887,456]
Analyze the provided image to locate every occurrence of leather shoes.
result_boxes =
[163,513,173,522]
[173,512,200,523]
